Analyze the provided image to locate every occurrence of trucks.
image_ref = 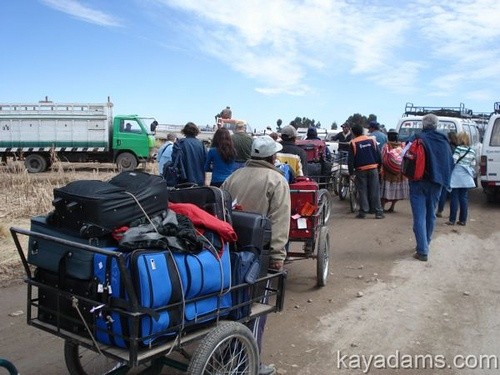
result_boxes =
[0,96,159,173]
[175,118,253,147]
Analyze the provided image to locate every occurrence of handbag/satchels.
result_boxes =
[164,161,177,187]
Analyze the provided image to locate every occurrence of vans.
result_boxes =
[480,101,500,203]
[395,102,480,198]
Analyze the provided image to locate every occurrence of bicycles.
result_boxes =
[348,174,361,212]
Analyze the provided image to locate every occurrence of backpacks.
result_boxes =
[400,132,427,181]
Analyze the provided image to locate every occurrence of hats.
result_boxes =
[341,122,350,128]
[369,121,379,129]
[281,125,299,138]
[251,135,283,158]
[386,129,398,135]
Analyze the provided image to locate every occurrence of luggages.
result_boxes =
[294,139,326,163]
[27,170,272,350]
[289,175,319,239]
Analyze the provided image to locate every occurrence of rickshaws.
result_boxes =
[8,226,288,375]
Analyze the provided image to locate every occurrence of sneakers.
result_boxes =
[259,362,276,375]
[375,209,385,219]
[357,211,368,218]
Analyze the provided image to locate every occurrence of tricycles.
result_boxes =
[331,150,350,201]
[280,175,332,287]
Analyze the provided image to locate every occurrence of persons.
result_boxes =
[305,126,331,163]
[124,123,132,132]
[172,122,207,188]
[380,129,409,212]
[151,121,159,135]
[436,132,458,217]
[156,132,177,177]
[220,106,232,119]
[280,125,308,177]
[231,120,254,170]
[203,127,237,187]
[347,124,385,219]
[368,120,388,150]
[218,135,292,375]
[445,132,476,225]
[270,132,278,142]
[404,114,455,260]
[332,123,352,151]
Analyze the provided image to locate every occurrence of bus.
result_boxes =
[471,113,490,150]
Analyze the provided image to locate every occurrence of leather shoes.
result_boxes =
[411,251,427,261]
